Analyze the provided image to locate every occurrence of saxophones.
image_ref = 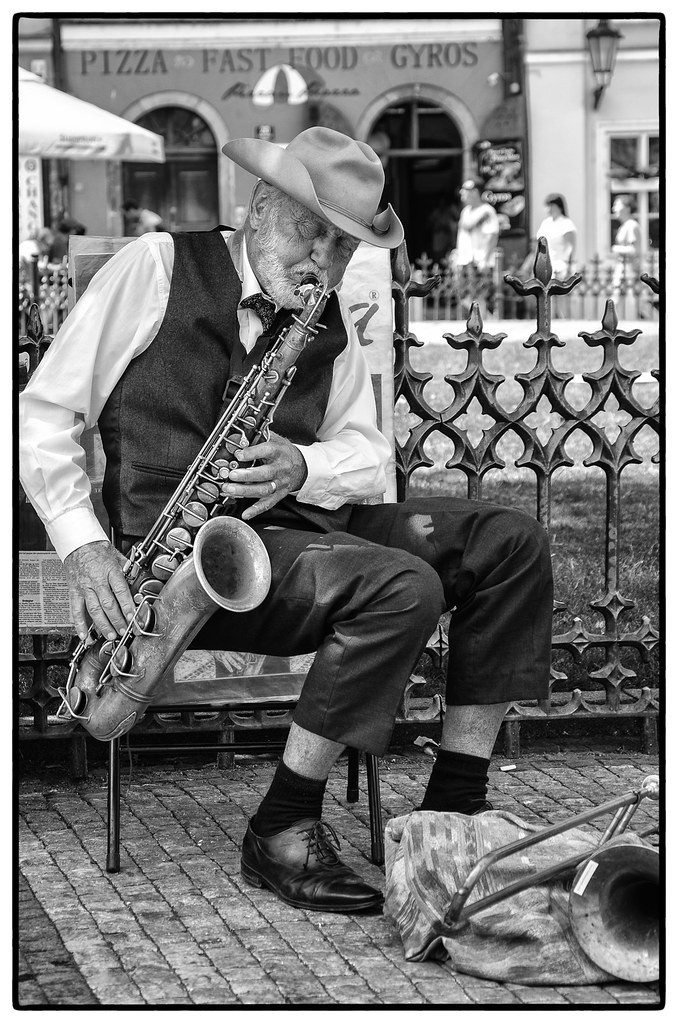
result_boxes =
[54,268,335,744]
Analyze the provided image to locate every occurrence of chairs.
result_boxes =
[104,524,384,870]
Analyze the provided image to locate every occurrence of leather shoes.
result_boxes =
[240,814,385,912]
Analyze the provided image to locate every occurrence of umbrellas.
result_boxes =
[18,66,166,163]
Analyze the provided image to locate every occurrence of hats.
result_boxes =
[223,125,406,249]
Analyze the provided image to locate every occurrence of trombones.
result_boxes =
[442,775,661,988]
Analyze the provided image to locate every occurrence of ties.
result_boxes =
[242,292,277,334]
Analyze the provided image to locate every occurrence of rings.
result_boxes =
[269,481,276,494]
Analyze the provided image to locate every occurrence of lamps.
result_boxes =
[586,18,623,107]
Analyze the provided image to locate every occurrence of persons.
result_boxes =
[120,198,164,237]
[19,127,553,912]
[19,217,86,262]
[456,178,500,311]
[608,195,642,318]
[536,193,577,275]
